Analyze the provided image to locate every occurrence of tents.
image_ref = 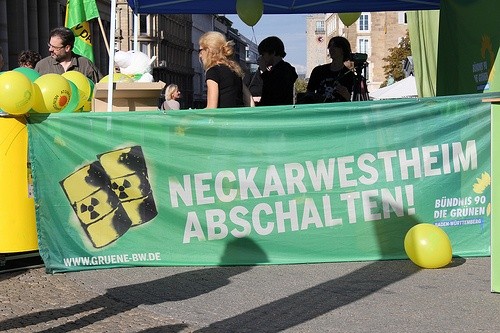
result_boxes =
[109,0,500,113]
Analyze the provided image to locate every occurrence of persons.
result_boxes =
[17,49,41,69]
[197,31,254,108]
[36,26,104,83]
[306,36,355,101]
[160,84,181,110]
[255,36,299,106]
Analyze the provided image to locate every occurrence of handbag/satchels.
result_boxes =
[248,69,263,96]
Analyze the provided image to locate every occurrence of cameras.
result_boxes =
[348,53,367,63]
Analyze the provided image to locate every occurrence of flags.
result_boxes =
[64,0,100,63]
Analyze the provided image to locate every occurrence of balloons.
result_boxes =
[235,0,264,27]
[403,223,453,269]
[338,12,362,27]
[0,67,133,114]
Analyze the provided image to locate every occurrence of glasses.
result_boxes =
[47,43,66,51]
[198,48,204,54]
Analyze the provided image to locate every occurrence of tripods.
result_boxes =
[345,62,370,102]
